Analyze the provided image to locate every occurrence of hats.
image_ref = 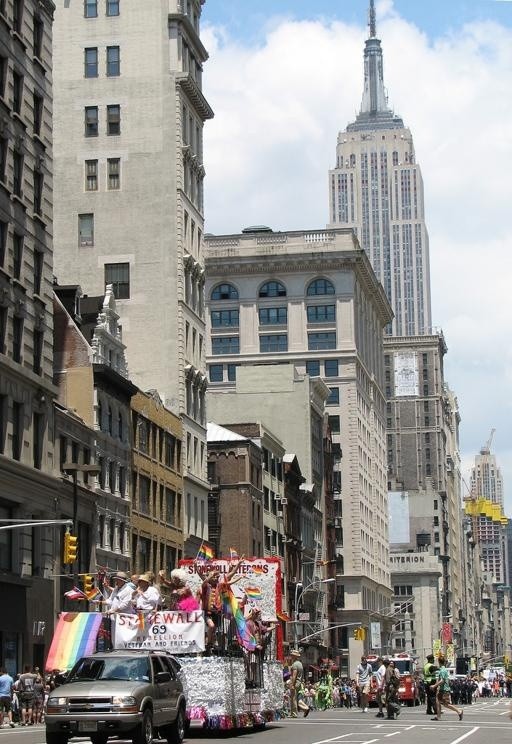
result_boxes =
[111,571,128,584]
[289,649,301,657]
[128,571,156,585]
[427,654,435,659]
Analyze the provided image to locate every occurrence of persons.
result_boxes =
[0,663,66,729]
[284,649,512,720]
[91,568,279,689]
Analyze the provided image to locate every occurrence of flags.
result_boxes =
[196,544,215,563]
[65,586,87,600]
[85,587,104,605]
[276,612,289,622]
[230,547,237,557]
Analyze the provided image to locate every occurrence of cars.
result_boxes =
[44,648,187,744]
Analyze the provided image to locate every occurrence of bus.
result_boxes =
[366,653,420,707]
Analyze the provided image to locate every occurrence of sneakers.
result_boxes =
[375,711,395,720]
[286,715,297,718]
[303,709,310,717]
[426,711,443,721]
[0,720,41,728]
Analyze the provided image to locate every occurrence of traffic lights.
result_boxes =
[63,535,79,564]
[360,628,366,640]
[503,656,507,664]
[84,575,94,595]
[353,627,359,640]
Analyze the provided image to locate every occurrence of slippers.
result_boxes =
[458,710,463,720]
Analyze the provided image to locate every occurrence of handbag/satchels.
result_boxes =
[391,669,400,687]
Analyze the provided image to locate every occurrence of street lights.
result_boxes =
[62,462,102,589]
[295,577,336,649]
